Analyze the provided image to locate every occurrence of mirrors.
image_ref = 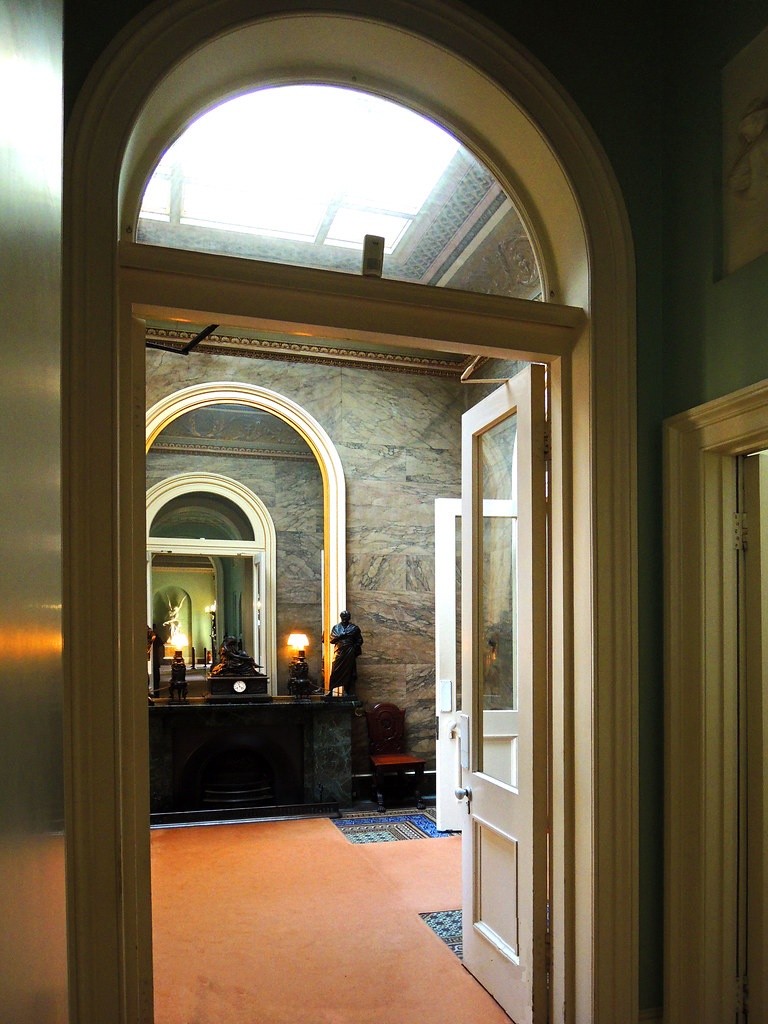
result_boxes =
[145,382,348,704]
[144,471,278,696]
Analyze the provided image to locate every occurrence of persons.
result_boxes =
[153,624,165,698]
[219,636,262,671]
[163,606,181,643]
[147,625,157,661]
[326,610,364,696]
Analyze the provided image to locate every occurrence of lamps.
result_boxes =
[167,630,188,701]
[288,632,313,697]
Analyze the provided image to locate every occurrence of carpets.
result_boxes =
[419,908,463,962]
[329,805,460,843]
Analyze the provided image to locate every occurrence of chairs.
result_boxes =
[363,702,427,812]
